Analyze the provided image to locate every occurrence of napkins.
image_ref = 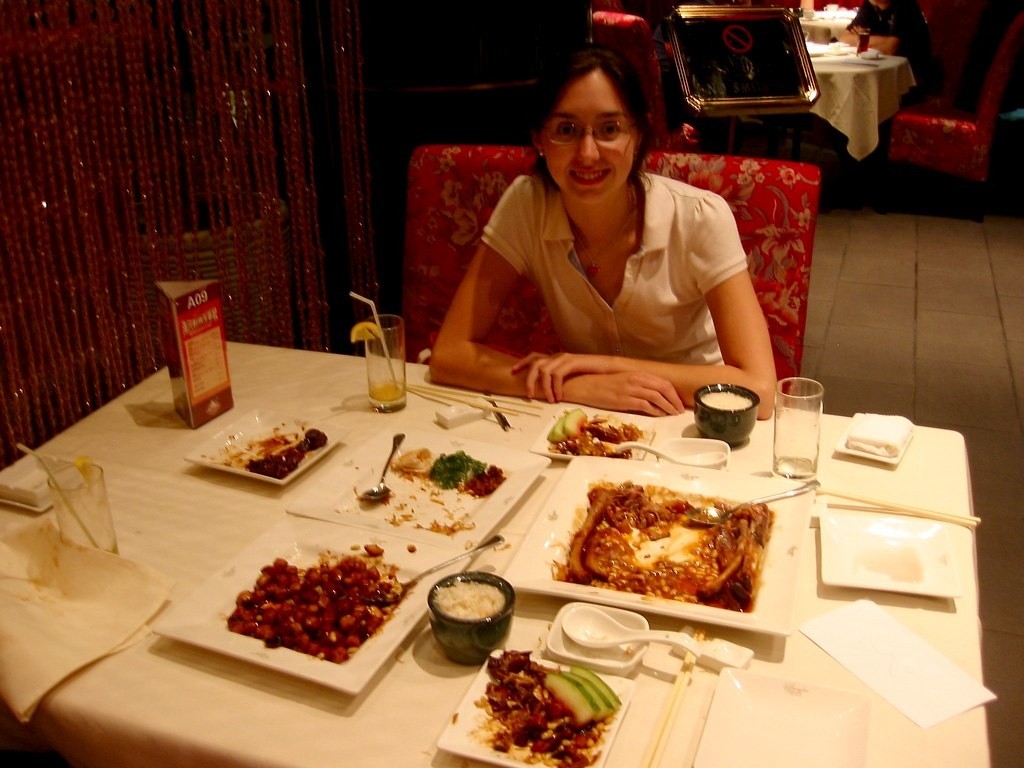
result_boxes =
[846,412,916,457]
[796,595,997,728]
[0,517,168,723]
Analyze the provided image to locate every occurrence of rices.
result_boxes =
[434,578,505,619]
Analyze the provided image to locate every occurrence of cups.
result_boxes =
[362,314,407,414]
[769,377,824,483]
[47,462,119,556]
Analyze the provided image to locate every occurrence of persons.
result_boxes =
[654,0,717,97]
[823,1,933,167]
[428,41,776,419]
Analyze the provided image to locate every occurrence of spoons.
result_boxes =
[686,479,821,525]
[615,441,727,470]
[359,432,406,501]
[562,606,702,659]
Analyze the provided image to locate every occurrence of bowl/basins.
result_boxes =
[693,383,761,447]
[425,570,517,666]
[547,602,650,676]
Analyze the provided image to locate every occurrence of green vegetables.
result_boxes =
[430,451,488,490]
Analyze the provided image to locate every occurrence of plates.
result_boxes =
[152,514,472,696]
[183,407,352,487]
[820,508,976,599]
[0,478,83,512]
[530,410,658,460]
[833,413,914,464]
[285,426,552,554]
[436,648,637,768]
[503,454,816,637]
[692,666,873,768]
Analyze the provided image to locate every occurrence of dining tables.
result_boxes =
[800,12,859,44]
[0,342,990,768]
[803,46,915,210]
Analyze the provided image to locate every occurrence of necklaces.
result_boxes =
[572,204,637,273]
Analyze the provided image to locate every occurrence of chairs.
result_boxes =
[590,16,699,155]
[876,17,1024,222]
[911,1,984,104]
[408,142,820,405]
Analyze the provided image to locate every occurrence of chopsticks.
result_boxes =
[815,486,981,526]
[405,383,543,416]
[641,626,705,768]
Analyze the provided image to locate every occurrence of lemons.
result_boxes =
[76,456,93,477]
[351,322,377,342]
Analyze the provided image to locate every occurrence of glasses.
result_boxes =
[541,117,641,147]
